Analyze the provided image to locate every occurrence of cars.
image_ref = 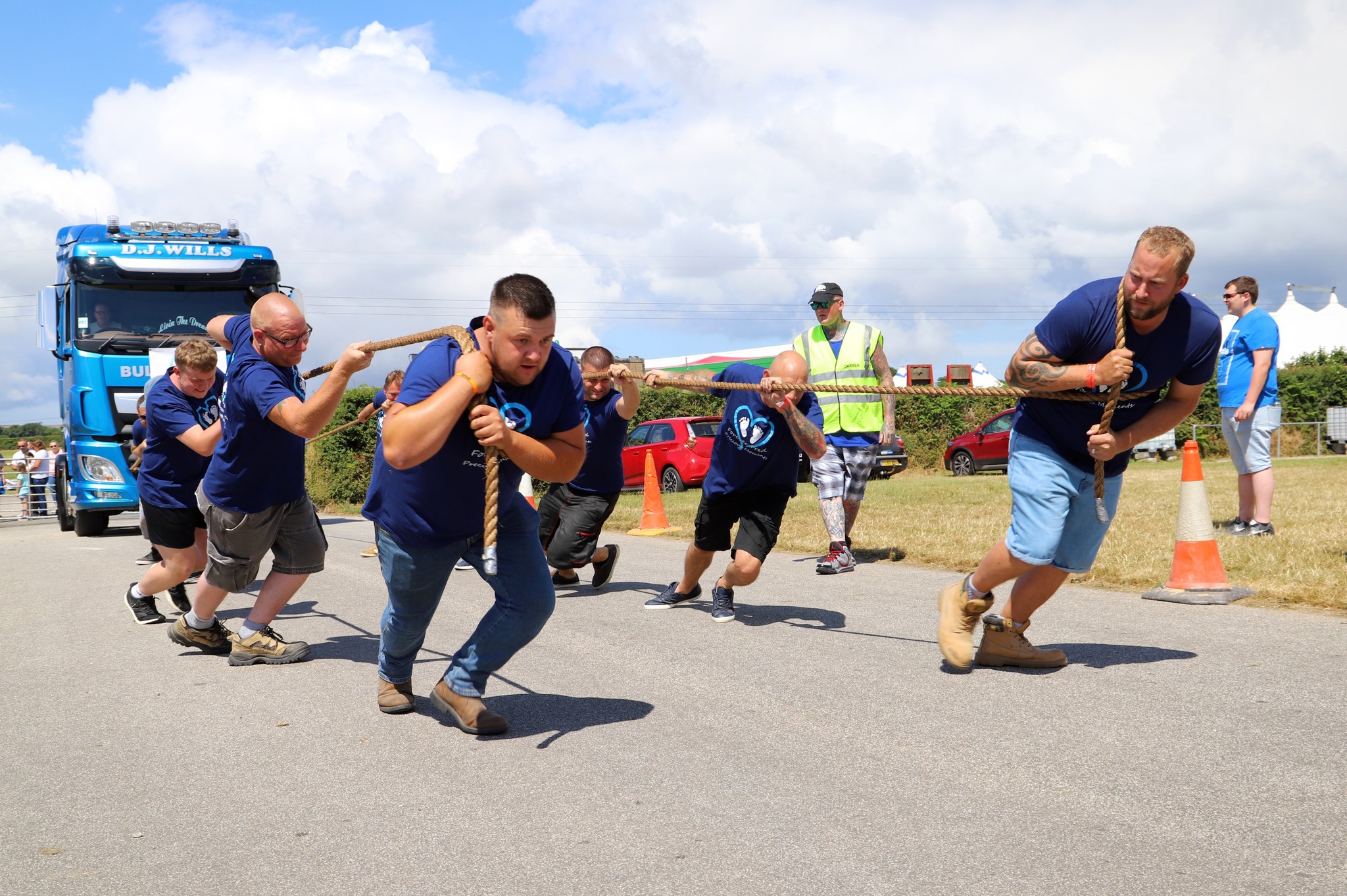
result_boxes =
[866,435,908,482]
[620,415,723,494]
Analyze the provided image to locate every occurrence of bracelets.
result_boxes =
[454,373,478,396]
[1086,364,1096,388]
[775,397,791,409]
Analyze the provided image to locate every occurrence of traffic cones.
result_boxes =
[626,449,683,537]
[517,471,536,511]
[1139,440,1253,605]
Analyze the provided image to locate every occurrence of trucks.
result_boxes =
[36,215,294,537]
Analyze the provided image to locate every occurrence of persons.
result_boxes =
[124,340,226,624]
[538,346,641,589]
[360,274,586,735]
[937,227,1222,670]
[794,283,897,574]
[135,394,164,565]
[167,293,374,666]
[0,439,64,521]
[357,370,404,558]
[1216,276,1280,536]
[645,351,827,622]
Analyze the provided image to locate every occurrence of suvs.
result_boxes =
[943,407,1017,476]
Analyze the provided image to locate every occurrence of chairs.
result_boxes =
[662,428,673,440]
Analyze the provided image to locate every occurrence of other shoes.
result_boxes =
[14,508,57,521]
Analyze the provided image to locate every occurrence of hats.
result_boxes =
[808,283,844,305]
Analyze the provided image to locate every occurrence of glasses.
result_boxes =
[94,310,111,315]
[17,445,25,448]
[810,300,839,310]
[50,445,57,448]
[259,323,313,348]
[138,415,147,420]
[1223,292,1253,300]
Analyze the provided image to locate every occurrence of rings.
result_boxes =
[1092,449,1096,454]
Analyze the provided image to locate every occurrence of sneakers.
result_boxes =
[845,536,856,565]
[712,575,735,622]
[973,612,1067,667]
[552,570,580,588]
[167,611,237,655]
[816,541,854,574]
[936,570,995,669]
[228,625,311,666]
[123,546,205,625]
[377,675,416,712]
[1230,518,1275,537]
[592,545,620,589]
[1214,516,1249,535]
[644,581,702,609]
[454,559,475,570]
[360,543,378,557]
[429,676,510,733]
